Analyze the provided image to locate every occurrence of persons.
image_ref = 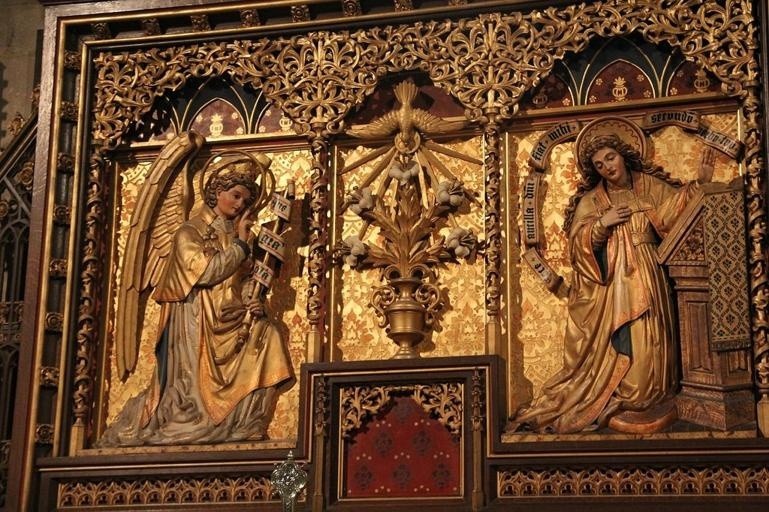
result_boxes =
[147,167,293,444]
[554,131,717,432]
